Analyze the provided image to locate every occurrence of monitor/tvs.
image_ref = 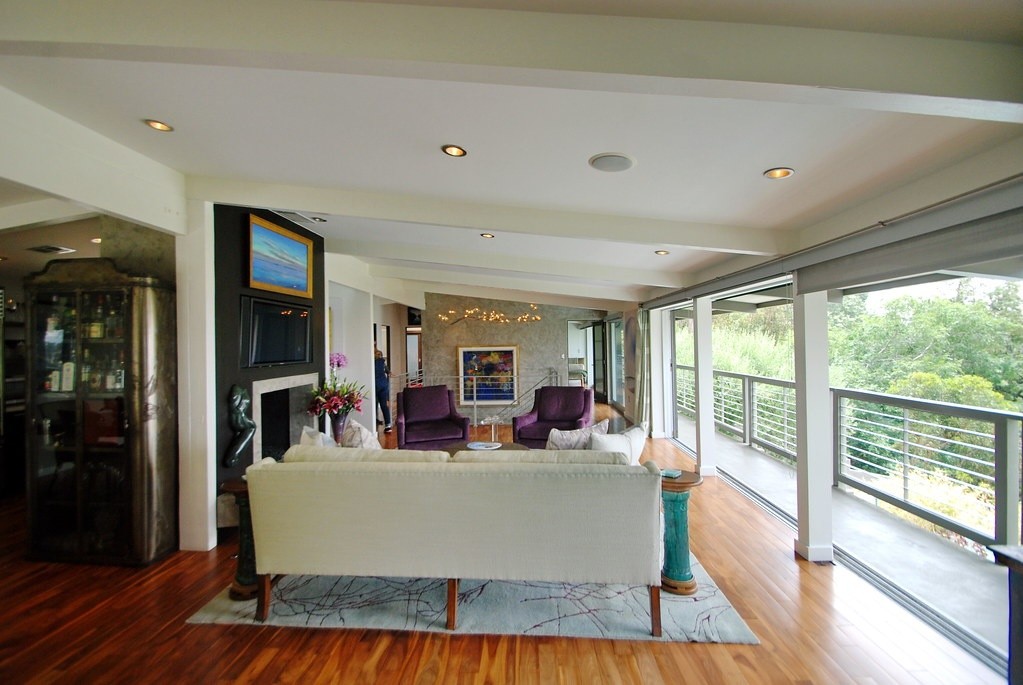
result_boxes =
[248,298,310,367]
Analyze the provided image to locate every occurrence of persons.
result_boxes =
[375,350,393,432]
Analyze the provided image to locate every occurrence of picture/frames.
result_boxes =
[456,344,521,407]
[249,213,314,301]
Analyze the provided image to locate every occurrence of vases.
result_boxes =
[331,412,348,445]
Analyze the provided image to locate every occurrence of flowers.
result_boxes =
[302,368,370,417]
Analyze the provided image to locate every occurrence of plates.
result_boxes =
[467,442,502,449]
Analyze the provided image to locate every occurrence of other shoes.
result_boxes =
[384,426,392,433]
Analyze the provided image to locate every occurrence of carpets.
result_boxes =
[186,548,760,644]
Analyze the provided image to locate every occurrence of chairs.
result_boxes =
[396,385,470,450]
[511,387,596,450]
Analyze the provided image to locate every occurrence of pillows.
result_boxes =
[299,426,337,447]
[543,419,610,450]
[341,418,382,449]
[591,420,649,466]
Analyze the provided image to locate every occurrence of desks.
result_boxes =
[442,440,531,457]
[660,469,705,594]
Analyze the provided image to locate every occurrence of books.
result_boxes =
[661,469,682,478]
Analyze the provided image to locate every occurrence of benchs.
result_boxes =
[246,444,665,636]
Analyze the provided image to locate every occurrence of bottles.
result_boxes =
[38,292,126,393]
